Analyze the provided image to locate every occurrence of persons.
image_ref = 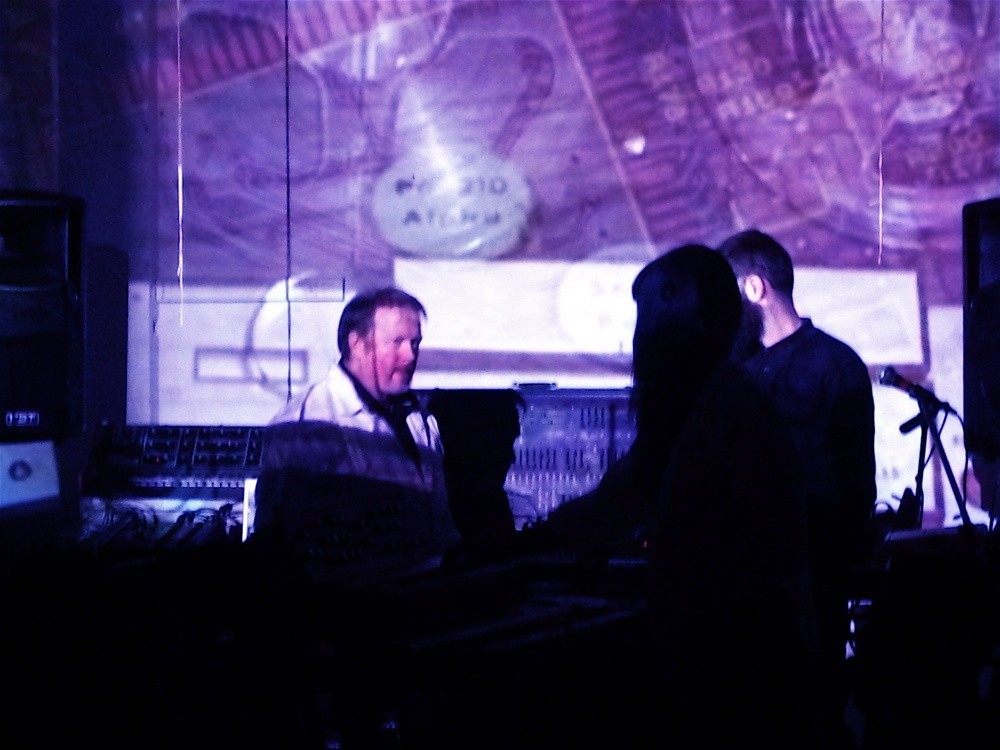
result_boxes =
[630,228,876,750]
[265,289,441,453]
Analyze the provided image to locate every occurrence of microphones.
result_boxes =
[881,364,956,414]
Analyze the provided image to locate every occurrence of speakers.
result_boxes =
[875,524,1000,750]
[0,196,84,434]
[960,197,1000,458]
